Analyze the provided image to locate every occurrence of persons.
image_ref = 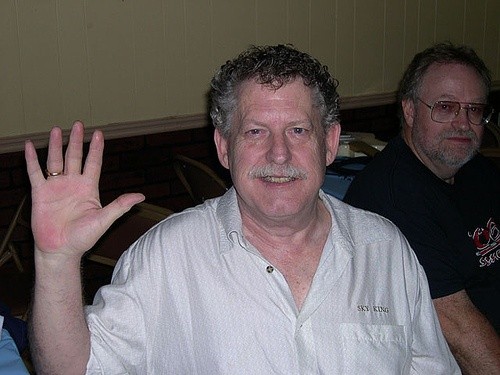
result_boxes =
[343,45,500,374]
[0,315,30,375]
[24,43,463,375]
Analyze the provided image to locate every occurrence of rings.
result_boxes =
[45,169,61,176]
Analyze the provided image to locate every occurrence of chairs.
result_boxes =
[171,154,229,206]
[81,203,173,266]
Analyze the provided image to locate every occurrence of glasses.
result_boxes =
[416,96,495,126]
